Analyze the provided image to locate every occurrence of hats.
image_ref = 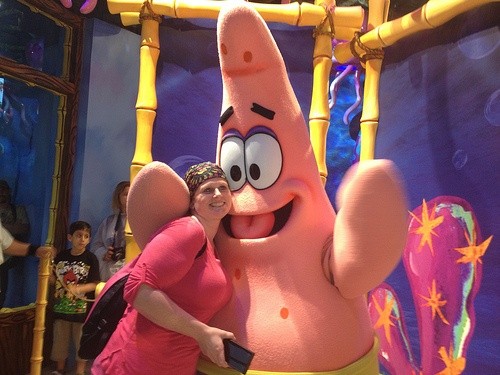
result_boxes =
[185,162,230,200]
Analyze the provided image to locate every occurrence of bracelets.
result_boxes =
[27,242,41,257]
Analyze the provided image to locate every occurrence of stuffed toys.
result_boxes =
[126,0,407,375]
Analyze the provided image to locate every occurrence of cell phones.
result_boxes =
[223,339,255,375]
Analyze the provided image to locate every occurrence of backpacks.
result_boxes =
[77,215,208,360]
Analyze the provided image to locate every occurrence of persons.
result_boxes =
[0,179,56,309]
[89,181,131,282]
[49,221,101,375]
[90,164,237,375]
